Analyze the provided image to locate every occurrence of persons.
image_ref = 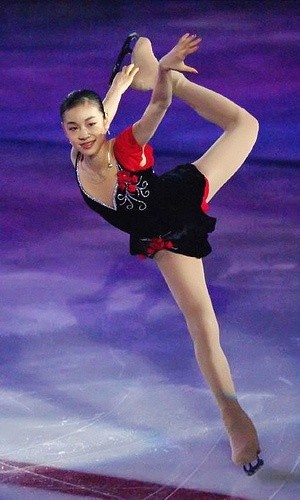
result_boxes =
[60,30,261,476]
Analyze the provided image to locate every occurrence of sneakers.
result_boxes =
[109,33,153,91]
[223,409,264,476]
